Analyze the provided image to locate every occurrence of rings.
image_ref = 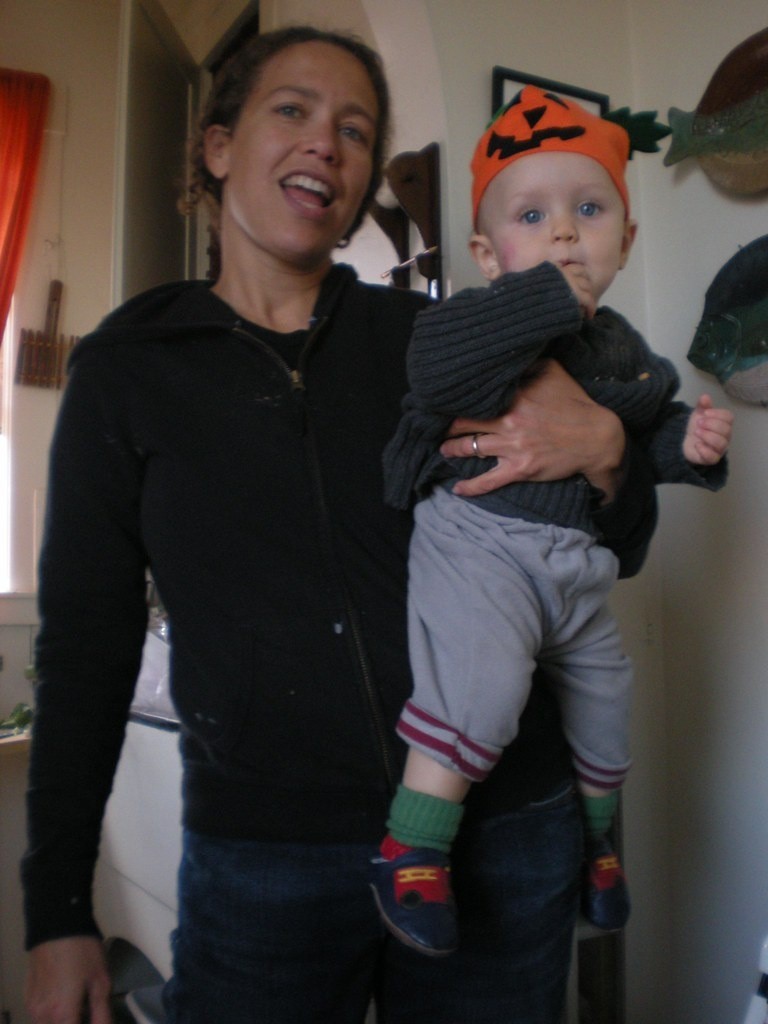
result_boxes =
[473,433,486,458]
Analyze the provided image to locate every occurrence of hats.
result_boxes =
[472,85,631,244]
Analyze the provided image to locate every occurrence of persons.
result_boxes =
[359,86,737,957]
[18,26,659,1024]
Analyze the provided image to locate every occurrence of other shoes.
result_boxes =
[582,838,631,934]
[359,833,460,956]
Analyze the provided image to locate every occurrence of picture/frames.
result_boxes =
[488,65,615,117]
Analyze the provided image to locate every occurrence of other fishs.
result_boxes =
[686,233,768,407]
[661,24,768,198]
